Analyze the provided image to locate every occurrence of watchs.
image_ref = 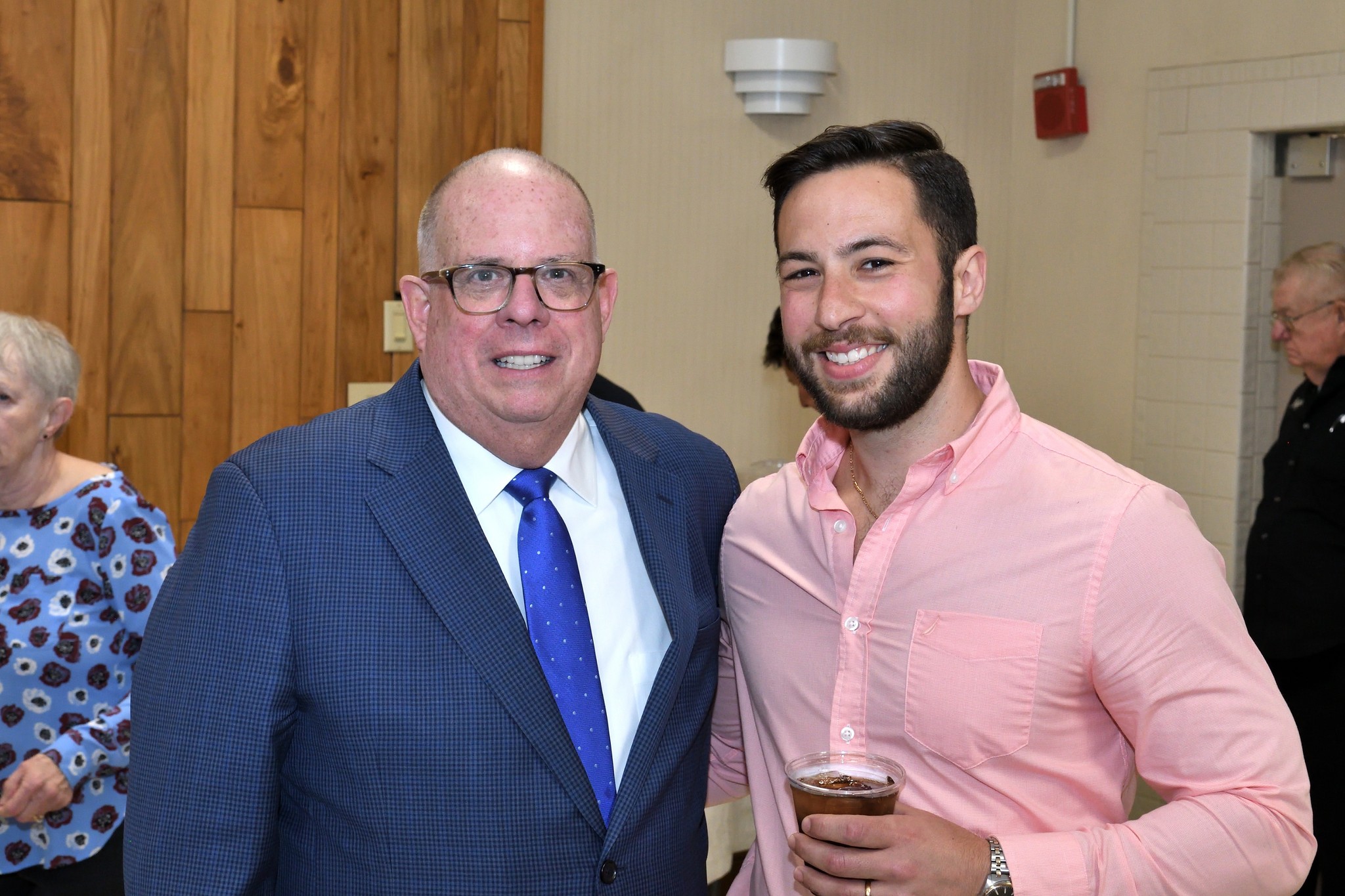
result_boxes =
[977,834,1017,896]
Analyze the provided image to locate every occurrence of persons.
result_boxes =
[696,116,1318,895]
[121,146,747,896]
[0,306,184,896]
[1242,241,1345,896]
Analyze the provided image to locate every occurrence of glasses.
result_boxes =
[420,261,606,314]
[1273,301,1334,330]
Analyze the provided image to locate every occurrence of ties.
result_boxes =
[508,466,616,828]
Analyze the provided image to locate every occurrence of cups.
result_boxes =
[783,751,905,896]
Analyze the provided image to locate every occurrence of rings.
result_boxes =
[862,879,873,896]
[35,812,44,825]
